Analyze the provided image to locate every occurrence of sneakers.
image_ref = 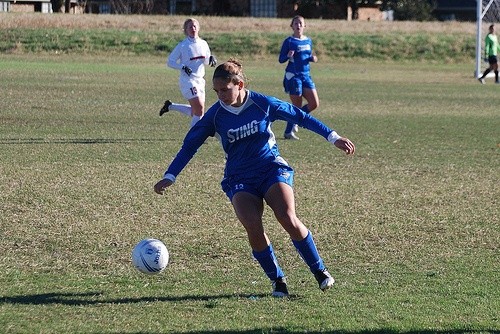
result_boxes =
[159,100,172,116]
[270,277,289,297]
[293,123,299,132]
[313,267,334,292]
[283,132,299,140]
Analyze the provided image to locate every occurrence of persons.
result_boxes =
[478,24,500,84]
[159,18,217,144]
[154,52,355,298]
[279,15,319,141]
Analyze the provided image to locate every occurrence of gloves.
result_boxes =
[209,56,216,67]
[184,66,192,77]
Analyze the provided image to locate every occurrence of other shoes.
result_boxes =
[478,78,484,84]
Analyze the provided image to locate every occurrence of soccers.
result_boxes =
[131,238,169,276]
